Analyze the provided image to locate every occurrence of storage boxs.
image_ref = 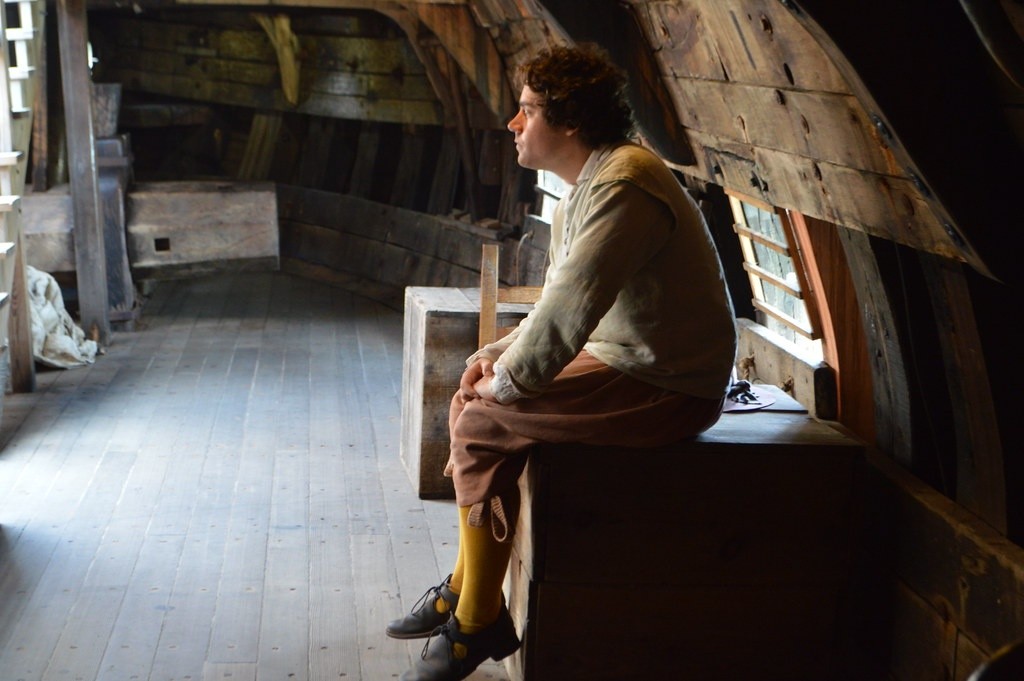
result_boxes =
[400,284,536,499]
[503,385,869,681]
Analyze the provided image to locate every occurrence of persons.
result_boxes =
[386,46,738,681]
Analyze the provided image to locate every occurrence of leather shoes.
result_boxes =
[385,575,457,640]
[398,604,520,681]
